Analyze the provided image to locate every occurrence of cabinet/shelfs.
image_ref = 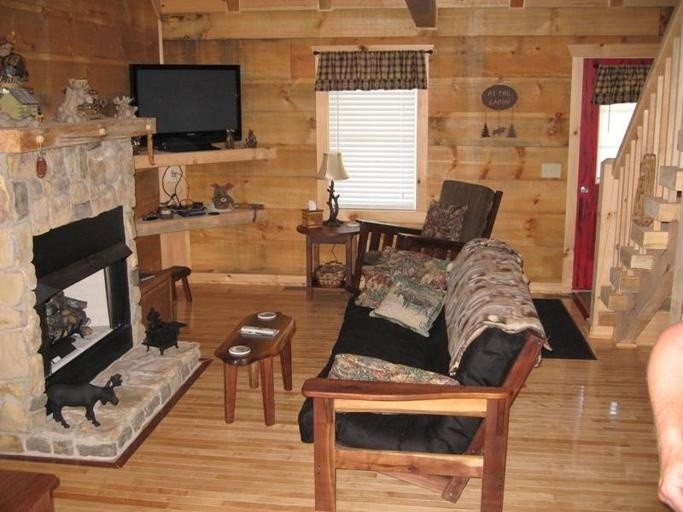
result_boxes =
[1,469,64,512]
[139,270,173,324]
[0,116,158,157]
[132,147,277,239]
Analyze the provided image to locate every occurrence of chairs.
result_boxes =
[354,179,503,295]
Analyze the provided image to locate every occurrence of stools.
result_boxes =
[163,266,192,302]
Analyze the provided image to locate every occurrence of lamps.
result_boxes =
[316,151,347,228]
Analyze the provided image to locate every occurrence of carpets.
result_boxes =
[531,298,599,360]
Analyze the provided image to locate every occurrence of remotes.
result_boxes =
[240,325,279,337]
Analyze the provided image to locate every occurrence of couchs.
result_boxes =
[301,235,544,511]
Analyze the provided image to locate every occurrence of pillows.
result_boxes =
[371,277,445,338]
[421,198,467,242]
[351,247,449,310]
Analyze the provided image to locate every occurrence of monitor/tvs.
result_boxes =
[128,63,242,150]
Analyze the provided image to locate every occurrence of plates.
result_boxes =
[257,312,277,320]
[228,345,251,356]
